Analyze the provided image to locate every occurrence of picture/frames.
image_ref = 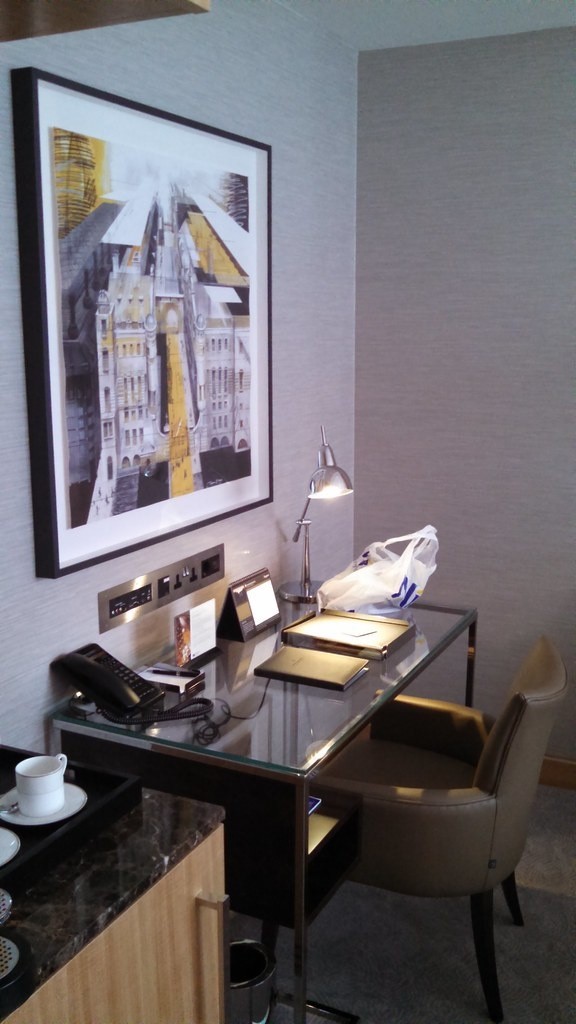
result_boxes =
[10,66,273,579]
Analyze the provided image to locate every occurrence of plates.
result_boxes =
[0,781,88,825]
[0,827,21,867]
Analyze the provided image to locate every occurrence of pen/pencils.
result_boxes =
[146,669,198,677]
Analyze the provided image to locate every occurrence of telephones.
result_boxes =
[49,643,167,720]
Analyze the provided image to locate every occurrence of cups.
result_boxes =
[15,753,68,817]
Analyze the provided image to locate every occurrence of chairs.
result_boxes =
[261,635,567,1024]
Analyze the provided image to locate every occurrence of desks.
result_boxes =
[49,583,475,1024]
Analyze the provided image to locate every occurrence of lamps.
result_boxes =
[275,424,354,604]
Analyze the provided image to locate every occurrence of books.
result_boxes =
[254,646,369,690]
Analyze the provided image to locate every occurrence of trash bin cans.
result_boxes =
[227,936,275,1024]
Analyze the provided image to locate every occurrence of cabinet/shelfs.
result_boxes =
[0,743,228,1024]
